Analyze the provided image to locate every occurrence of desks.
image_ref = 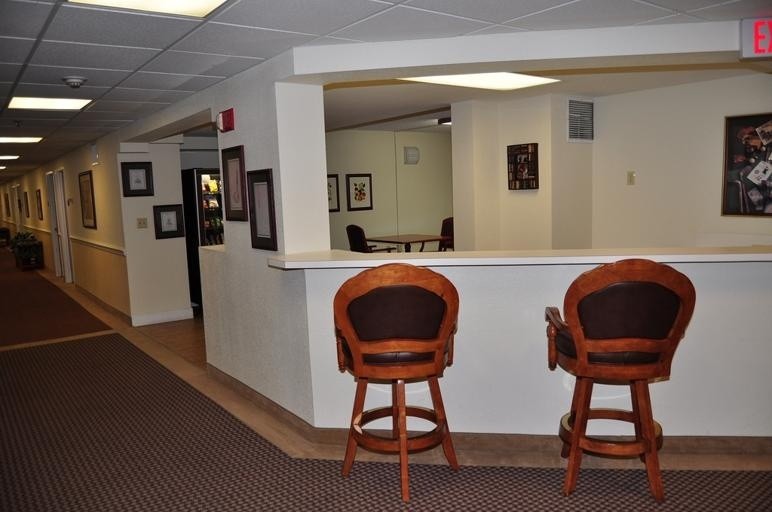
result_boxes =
[367,234,452,252]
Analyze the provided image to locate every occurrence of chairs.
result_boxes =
[346,224,395,252]
[545,259,696,504]
[333,262,460,502]
[438,217,454,251]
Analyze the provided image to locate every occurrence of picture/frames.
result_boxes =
[120,161,154,198]
[78,170,97,229]
[327,174,340,212]
[221,145,249,222]
[246,168,278,251]
[152,204,185,239]
[4,189,43,220]
[346,174,373,212]
[720,112,772,218]
